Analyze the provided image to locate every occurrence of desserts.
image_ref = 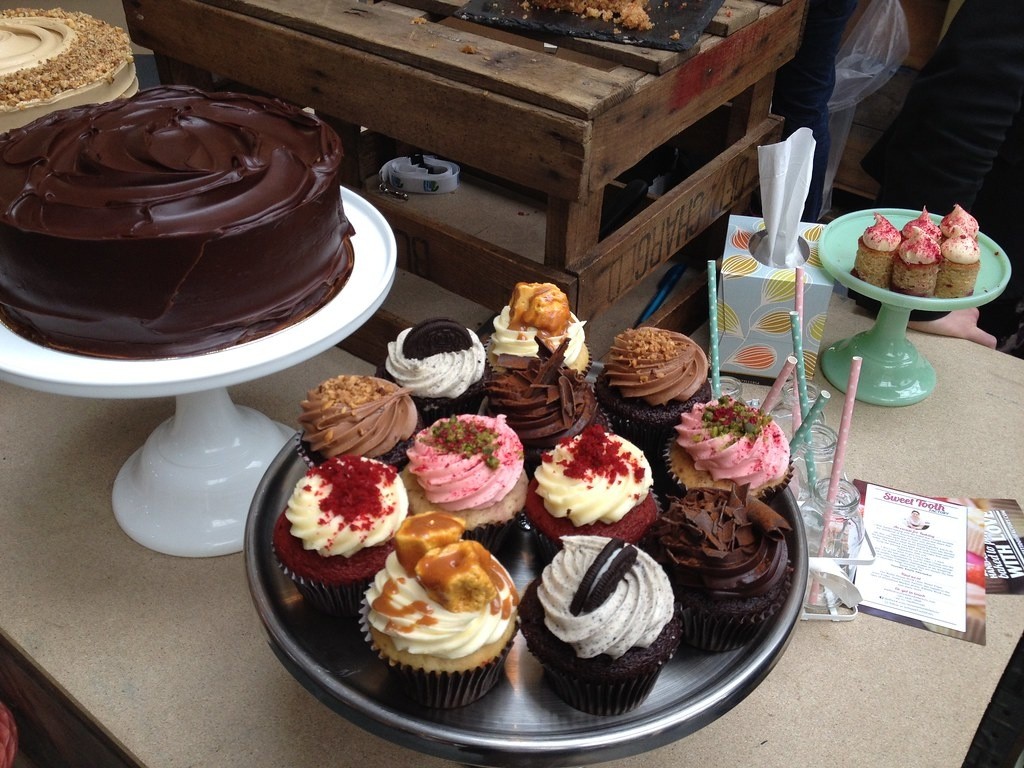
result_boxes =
[852,203,981,298]
[272,283,795,717]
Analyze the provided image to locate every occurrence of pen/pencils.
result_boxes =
[632,253,694,326]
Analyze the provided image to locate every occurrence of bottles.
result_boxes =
[770,379,826,444]
[709,376,748,408]
[791,424,849,507]
[799,478,866,612]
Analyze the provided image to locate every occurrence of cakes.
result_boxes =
[0,4,138,136]
[0,83,356,361]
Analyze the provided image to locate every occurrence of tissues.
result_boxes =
[707,125,836,381]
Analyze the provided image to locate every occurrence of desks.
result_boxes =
[0,271,1024,768]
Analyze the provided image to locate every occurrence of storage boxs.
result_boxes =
[123,0,809,373]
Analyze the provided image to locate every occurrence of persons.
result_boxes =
[772,0,858,223]
[857,0,1024,349]
[906,510,927,530]
